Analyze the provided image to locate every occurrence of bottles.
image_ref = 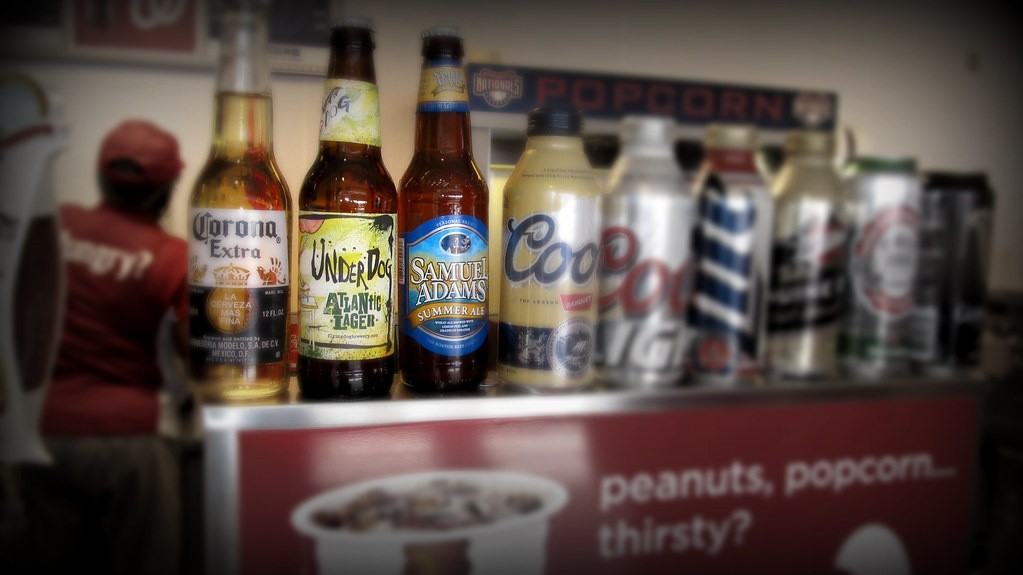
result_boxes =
[694,126,771,383]
[775,131,847,375]
[502,106,600,389]
[395,27,490,392]
[598,115,689,387]
[187,12,291,402]
[299,25,397,397]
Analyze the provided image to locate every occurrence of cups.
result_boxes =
[293,471,568,575]
